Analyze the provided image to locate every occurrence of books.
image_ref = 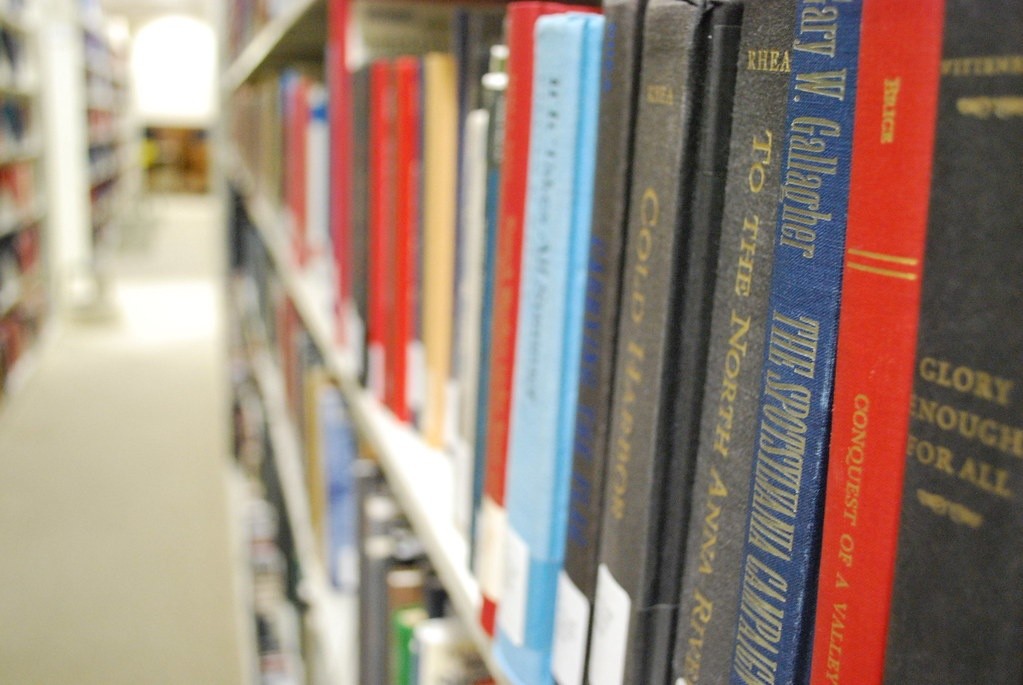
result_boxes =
[0,0,130,386]
[211,2,1021,684]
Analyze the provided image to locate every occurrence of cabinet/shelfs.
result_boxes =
[0,0,137,394]
[210,0,552,685]
